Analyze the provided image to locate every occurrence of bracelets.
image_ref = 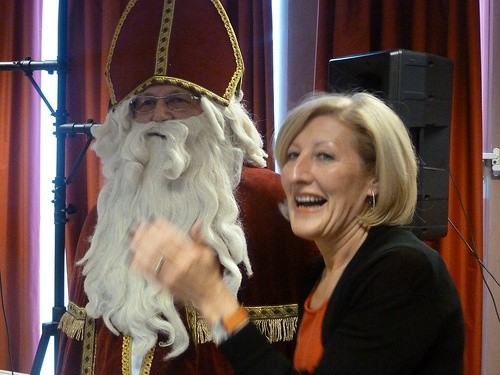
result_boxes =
[224,308,249,330]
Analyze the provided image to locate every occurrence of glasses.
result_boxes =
[130,92,200,113]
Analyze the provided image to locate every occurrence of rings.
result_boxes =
[155,258,165,274]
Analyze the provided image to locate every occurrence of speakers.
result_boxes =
[327,47,454,240]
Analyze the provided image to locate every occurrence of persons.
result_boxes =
[129,94,464,375]
[57,0,321,375]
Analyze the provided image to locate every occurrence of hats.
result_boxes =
[104,0,244,108]
